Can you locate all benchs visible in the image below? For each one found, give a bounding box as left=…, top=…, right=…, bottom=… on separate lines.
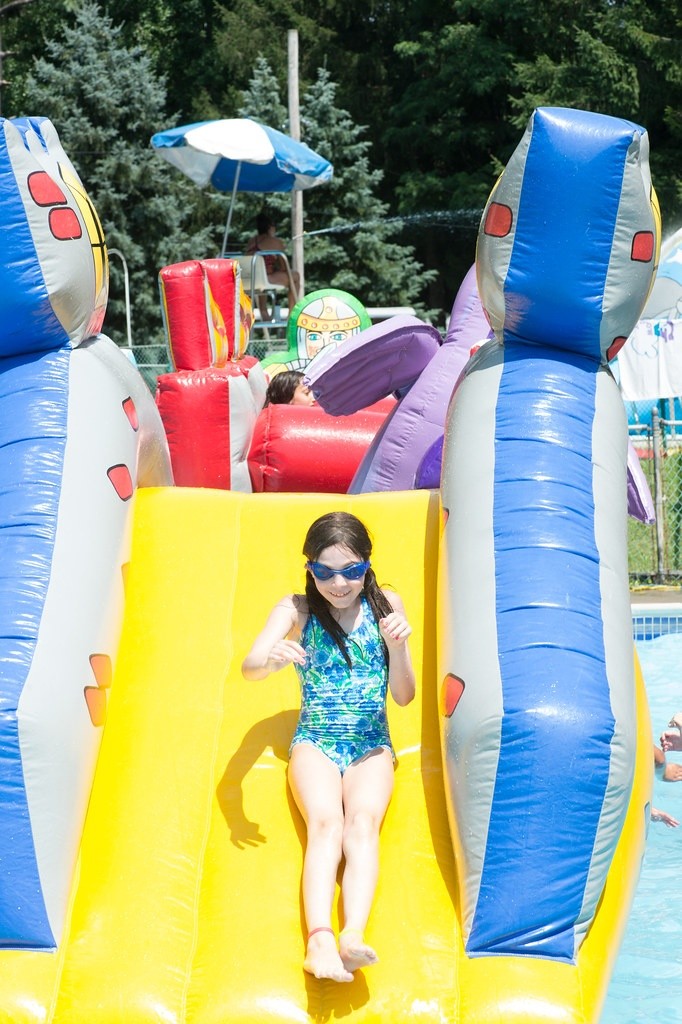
left=220, top=252, right=288, bottom=321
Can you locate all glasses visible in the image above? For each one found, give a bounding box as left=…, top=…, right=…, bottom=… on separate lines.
left=307, top=560, right=372, bottom=582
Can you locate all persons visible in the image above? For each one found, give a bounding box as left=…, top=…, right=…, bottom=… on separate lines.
left=262, top=370, right=315, bottom=407
left=246, top=215, right=301, bottom=338
left=650, top=714, right=682, bottom=828
left=242, top=511, right=415, bottom=982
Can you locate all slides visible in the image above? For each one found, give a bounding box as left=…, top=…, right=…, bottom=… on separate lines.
left=0, top=486, right=654, bottom=1024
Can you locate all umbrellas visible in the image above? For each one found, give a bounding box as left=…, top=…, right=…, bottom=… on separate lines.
left=151, top=118, right=334, bottom=258
